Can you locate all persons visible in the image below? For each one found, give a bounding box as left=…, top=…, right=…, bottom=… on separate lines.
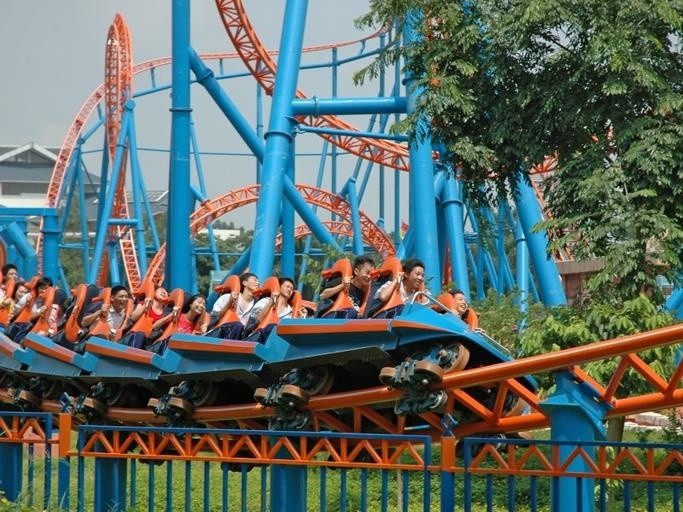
left=1, top=256, right=468, bottom=354
